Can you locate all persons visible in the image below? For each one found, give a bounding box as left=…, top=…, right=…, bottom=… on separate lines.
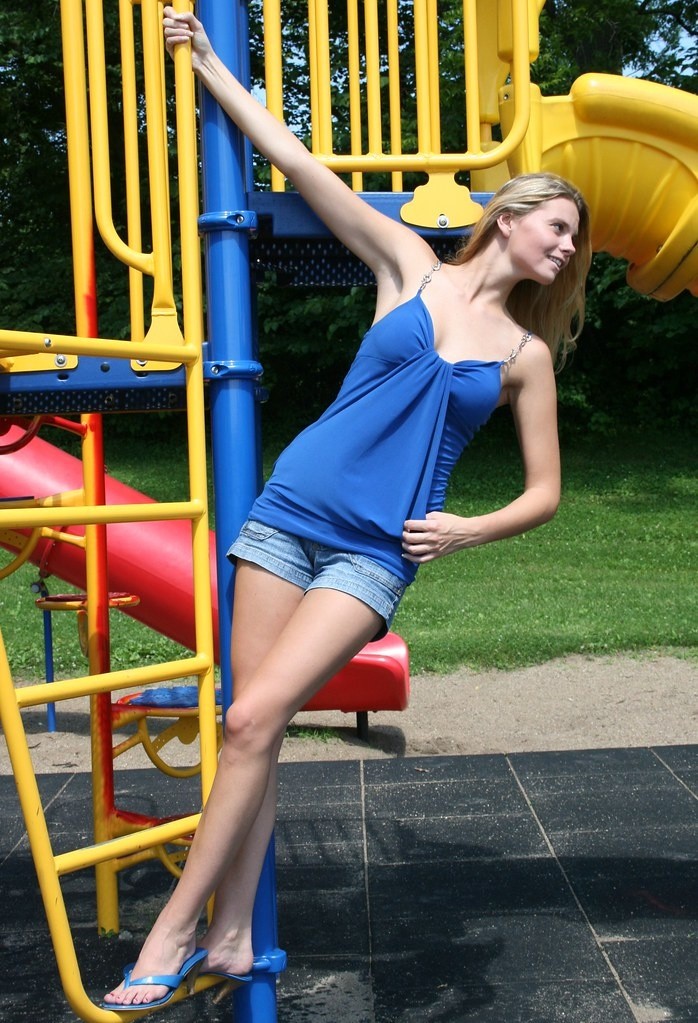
left=97, top=6, right=590, bottom=1010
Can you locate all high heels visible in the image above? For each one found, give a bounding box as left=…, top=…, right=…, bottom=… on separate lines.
left=100, top=945, right=210, bottom=1010
left=185, top=961, right=253, bottom=1005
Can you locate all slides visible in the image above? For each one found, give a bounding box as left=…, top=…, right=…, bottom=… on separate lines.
left=0, top=423, right=411, bottom=709
left=502, top=72, right=698, bottom=302
